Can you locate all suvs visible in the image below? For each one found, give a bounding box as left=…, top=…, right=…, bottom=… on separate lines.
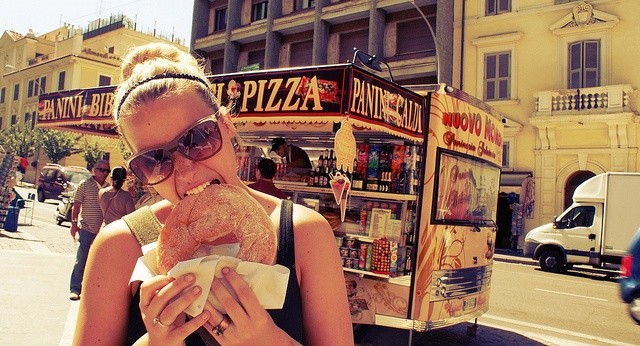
left=37, top=163, right=96, bottom=203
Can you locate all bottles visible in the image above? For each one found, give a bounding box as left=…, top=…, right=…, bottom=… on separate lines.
left=360, top=207, right=367, bottom=231
left=333, top=153, right=336, bottom=169
left=353, top=258, right=359, bottom=269
left=365, top=245, right=372, bottom=272
left=328, top=150, right=333, bottom=169
left=399, top=166, right=406, bottom=193
left=379, top=172, right=385, bottom=192
left=341, top=257, right=346, bottom=268
left=346, top=258, right=353, bottom=269
left=340, top=247, right=350, bottom=257
left=319, top=168, right=323, bottom=186
left=366, top=177, right=379, bottom=191
left=385, top=171, right=391, bottom=193
left=346, top=238, right=354, bottom=248
left=359, top=244, right=367, bottom=270
left=406, top=165, right=415, bottom=194
left=416, top=145, right=420, bottom=169
left=336, top=237, right=344, bottom=247
left=382, top=172, right=388, bottom=192
left=414, top=176, right=418, bottom=191
left=352, top=172, right=364, bottom=191
left=323, top=167, right=328, bottom=186
left=315, top=167, right=319, bottom=186
left=323, top=149, right=328, bottom=169
left=318, top=150, right=323, bottom=169
left=309, top=166, right=314, bottom=186
left=391, top=170, right=398, bottom=193
left=410, top=147, right=416, bottom=170
left=403, top=146, right=410, bottom=170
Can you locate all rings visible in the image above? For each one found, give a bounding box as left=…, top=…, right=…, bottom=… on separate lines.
left=153, top=317, right=170, bottom=329
left=212, top=316, right=229, bottom=335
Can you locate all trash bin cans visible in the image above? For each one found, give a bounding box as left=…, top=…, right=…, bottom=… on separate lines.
left=4, top=206, right=20, bottom=232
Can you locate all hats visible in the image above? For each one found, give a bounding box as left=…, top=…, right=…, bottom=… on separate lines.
left=271, top=138, right=285, bottom=151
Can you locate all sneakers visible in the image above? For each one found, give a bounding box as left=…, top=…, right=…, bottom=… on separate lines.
left=69, top=291, right=80, bottom=299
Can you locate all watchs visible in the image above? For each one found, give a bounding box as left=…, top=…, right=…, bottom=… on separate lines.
left=72, top=219, right=78, bottom=223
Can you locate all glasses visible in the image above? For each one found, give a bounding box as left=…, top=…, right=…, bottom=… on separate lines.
left=96, top=168, right=111, bottom=173
left=129, top=110, right=223, bottom=186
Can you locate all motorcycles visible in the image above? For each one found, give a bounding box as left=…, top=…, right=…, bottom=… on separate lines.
left=56, top=179, right=86, bottom=227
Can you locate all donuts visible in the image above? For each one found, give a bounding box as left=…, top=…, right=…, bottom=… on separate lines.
left=158, top=183, right=277, bottom=285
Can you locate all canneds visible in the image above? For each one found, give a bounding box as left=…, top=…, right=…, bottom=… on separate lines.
left=353, top=259, right=359, bottom=269
left=340, top=258, right=345, bottom=267
left=347, top=258, right=353, bottom=268
left=335, top=238, right=342, bottom=248
left=340, top=248, right=349, bottom=257
left=347, top=238, right=355, bottom=248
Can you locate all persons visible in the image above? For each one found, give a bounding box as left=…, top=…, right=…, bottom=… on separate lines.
left=69, top=160, right=111, bottom=300
left=97, top=167, right=136, bottom=226
left=269, top=138, right=312, bottom=169
left=246, top=158, right=287, bottom=200
left=72, top=42, right=354, bottom=346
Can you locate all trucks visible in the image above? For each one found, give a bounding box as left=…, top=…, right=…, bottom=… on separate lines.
left=523, top=172, right=639, bottom=279
